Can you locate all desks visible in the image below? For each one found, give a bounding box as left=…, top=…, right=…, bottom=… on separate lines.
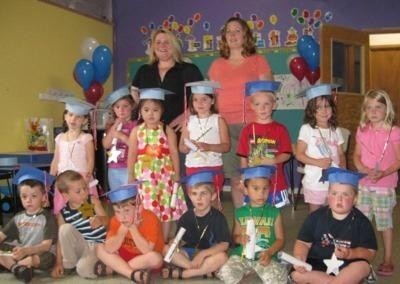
left=0, top=164, right=51, bottom=226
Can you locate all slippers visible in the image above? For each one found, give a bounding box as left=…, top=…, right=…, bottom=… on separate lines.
left=376, top=258, right=395, bottom=276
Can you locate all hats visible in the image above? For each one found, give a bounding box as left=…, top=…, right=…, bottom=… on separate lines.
left=99, top=184, right=140, bottom=225
left=244, top=80, right=282, bottom=95
left=104, top=85, right=130, bottom=106
left=294, top=83, right=342, bottom=109
left=177, top=170, right=224, bottom=211
left=236, top=165, right=277, bottom=206
left=57, top=96, right=97, bottom=151
left=134, top=87, right=175, bottom=120
left=11, top=166, right=55, bottom=209
left=318, top=167, right=368, bottom=184
left=182, top=80, right=222, bottom=126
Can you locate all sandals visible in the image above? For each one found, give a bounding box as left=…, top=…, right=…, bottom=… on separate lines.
left=11, top=265, right=34, bottom=284
left=129, top=268, right=151, bottom=284
left=160, top=265, right=184, bottom=280
left=95, top=260, right=117, bottom=277
left=202, top=272, right=217, bottom=279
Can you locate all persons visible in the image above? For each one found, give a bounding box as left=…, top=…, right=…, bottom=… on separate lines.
left=0, top=79, right=400, bottom=284
left=207, top=17, right=275, bottom=249
left=130, top=28, right=214, bottom=209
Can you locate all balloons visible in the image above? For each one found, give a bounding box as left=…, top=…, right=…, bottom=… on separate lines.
left=73, top=38, right=114, bottom=104
left=290, top=35, right=321, bottom=85
